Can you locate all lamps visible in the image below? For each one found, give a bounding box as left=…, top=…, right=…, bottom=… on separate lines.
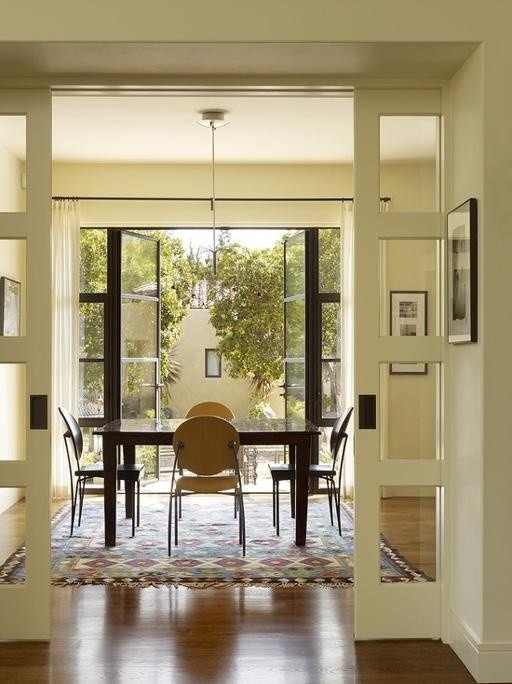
left=195, top=108, right=231, bottom=281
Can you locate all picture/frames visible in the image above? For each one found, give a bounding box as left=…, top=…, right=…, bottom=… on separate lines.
left=0, top=276, right=22, bottom=339
left=443, top=196, right=482, bottom=349
left=389, top=290, right=429, bottom=376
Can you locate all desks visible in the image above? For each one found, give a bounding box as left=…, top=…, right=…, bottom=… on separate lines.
left=86, top=415, right=323, bottom=551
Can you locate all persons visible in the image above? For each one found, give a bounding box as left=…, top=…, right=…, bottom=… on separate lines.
left=451, top=232, right=467, bottom=322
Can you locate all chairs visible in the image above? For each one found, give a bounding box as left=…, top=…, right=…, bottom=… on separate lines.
left=165, top=411, right=249, bottom=560
left=176, top=398, right=247, bottom=523
left=55, top=401, right=147, bottom=541
left=267, top=403, right=356, bottom=540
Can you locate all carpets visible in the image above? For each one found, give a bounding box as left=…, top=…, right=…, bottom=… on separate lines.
left=0, top=491, right=432, bottom=596
